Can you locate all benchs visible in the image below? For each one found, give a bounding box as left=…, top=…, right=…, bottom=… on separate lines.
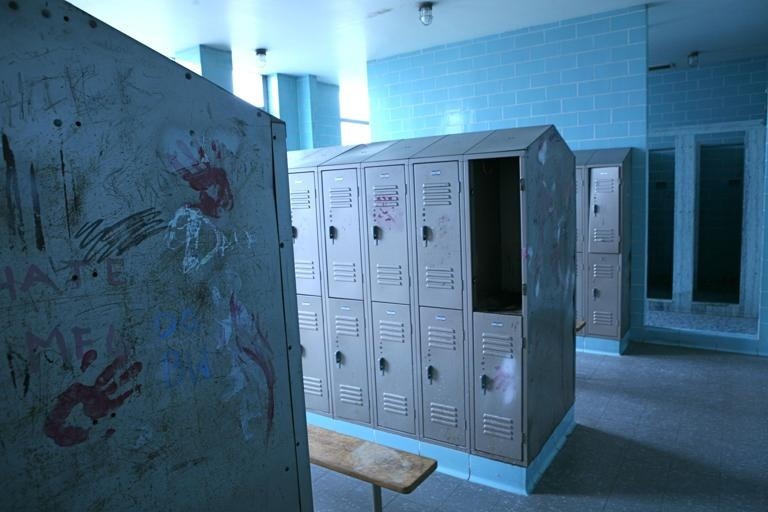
left=302, top=423, right=440, bottom=512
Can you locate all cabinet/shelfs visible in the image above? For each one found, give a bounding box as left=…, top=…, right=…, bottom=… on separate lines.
left=469, top=312, right=585, bottom=466
left=572, top=148, right=599, bottom=252
left=323, top=298, right=374, bottom=431
left=584, top=251, right=632, bottom=341
left=297, top=295, right=333, bottom=421
left=318, top=138, right=404, bottom=297
left=360, top=133, right=449, bottom=305
left=409, top=129, right=497, bottom=308
left=585, top=147, right=633, bottom=254
left=415, top=304, right=471, bottom=455
left=368, top=301, right=418, bottom=439
left=286, top=143, right=360, bottom=295
left=463, top=124, right=578, bottom=316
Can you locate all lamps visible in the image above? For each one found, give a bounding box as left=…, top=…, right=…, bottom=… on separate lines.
left=688, top=52, right=698, bottom=68
left=254, top=48, right=267, bottom=68
left=419, top=2, right=433, bottom=26
